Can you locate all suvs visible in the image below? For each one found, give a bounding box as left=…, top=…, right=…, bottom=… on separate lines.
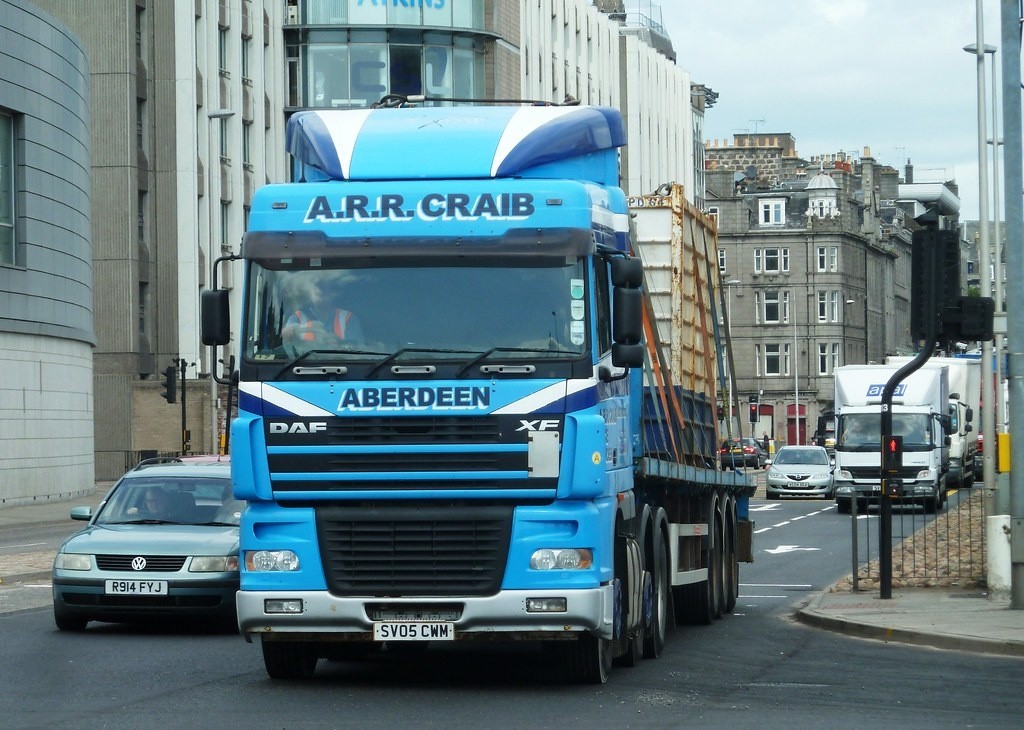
left=51, top=456, right=247, bottom=632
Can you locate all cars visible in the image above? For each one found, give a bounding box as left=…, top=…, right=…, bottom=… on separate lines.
left=171, top=456, right=231, bottom=505
left=719, top=437, right=768, bottom=470
left=811, top=428, right=836, bottom=456
left=764, top=445, right=836, bottom=500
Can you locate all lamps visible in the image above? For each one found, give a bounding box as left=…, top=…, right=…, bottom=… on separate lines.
left=207, top=108, right=236, bottom=121
left=845, top=299, right=855, bottom=306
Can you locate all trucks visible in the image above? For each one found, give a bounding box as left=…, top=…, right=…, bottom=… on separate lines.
left=833, top=364, right=952, bottom=514
left=951, top=354, right=1009, bottom=482
left=885, top=356, right=982, bottom=489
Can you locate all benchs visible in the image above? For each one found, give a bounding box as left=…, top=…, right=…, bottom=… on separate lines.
left=332, top=99, right=367, bottom=107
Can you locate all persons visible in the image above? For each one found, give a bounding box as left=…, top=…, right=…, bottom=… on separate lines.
left=762, top=430, right=769, bottom=444
left=213, top=484, right=247, bottom=524
left=780, top=450, right=823, bottom=465
left=281, top=275, right=367, bottom=350
left=848, top=420, right=868, bottom=441
left=127, top=487, right=178, bottom=522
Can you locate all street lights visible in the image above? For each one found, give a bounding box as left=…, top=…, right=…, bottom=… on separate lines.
left=208, top=108, right=236, bottom=456
left=964, top=43, right=1006, bottom=433
left=727, top=279, right=741, bottom=435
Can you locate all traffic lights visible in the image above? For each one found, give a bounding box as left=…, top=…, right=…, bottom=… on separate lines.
left=185, top=430, right=191, bottom=442
left=882, top=435, right=902, bottom=472
left=160, top=366, right=175, bottom=403
left=750, top=403, right=759, bottom=423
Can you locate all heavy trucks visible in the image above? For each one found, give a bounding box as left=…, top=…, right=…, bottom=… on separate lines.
left=203, top=92, right=758, bottom=686
